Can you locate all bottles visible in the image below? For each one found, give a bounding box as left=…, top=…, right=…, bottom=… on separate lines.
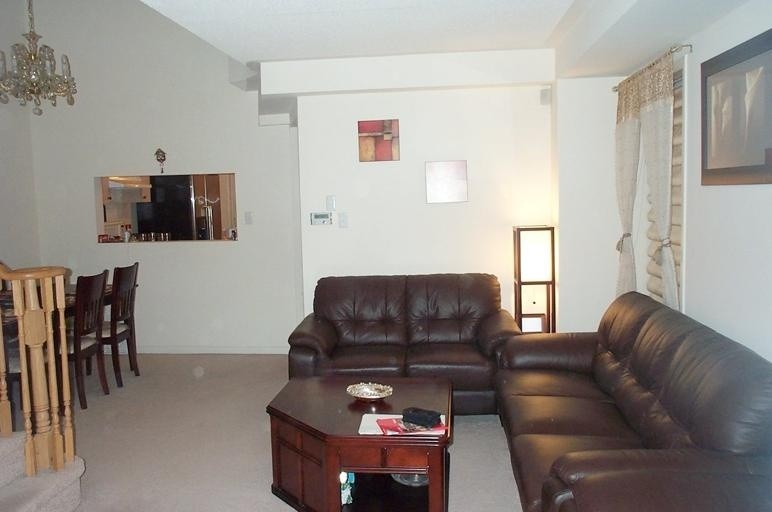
left=121, top=224, right=171, bottom=242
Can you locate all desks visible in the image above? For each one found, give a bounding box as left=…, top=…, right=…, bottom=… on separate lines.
left=0, top=283, right=139, bottom=337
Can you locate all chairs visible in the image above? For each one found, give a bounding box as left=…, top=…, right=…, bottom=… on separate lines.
left=6, top=278, right=66, bottom=432
left=55, top=269, right=110, bottom=410
left=87, top=262, right=140, bottom=387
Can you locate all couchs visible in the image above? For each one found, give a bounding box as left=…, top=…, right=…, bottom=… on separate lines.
left=493, top=291, right=772, bottom=512
left=288, top=273, right=523, bottom=416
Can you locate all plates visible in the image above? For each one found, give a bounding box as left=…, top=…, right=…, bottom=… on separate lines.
left=345, top=383, right=393, bottom=402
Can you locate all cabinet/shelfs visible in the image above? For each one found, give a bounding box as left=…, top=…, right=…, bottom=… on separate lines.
left=193, top=175, right=234, bottom=239
left=101, top=176, right=151, bottom=204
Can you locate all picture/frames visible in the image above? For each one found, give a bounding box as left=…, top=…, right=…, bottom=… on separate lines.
left=701, top=28, right=772, bottom=185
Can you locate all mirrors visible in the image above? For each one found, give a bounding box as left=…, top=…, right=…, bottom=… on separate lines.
left=93, top=173, right=238, bottom=243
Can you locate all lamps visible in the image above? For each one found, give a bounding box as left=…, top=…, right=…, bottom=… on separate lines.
left=513, top=225, right=556, bottom=334
left=0, top=0, right=77, bottom=115
